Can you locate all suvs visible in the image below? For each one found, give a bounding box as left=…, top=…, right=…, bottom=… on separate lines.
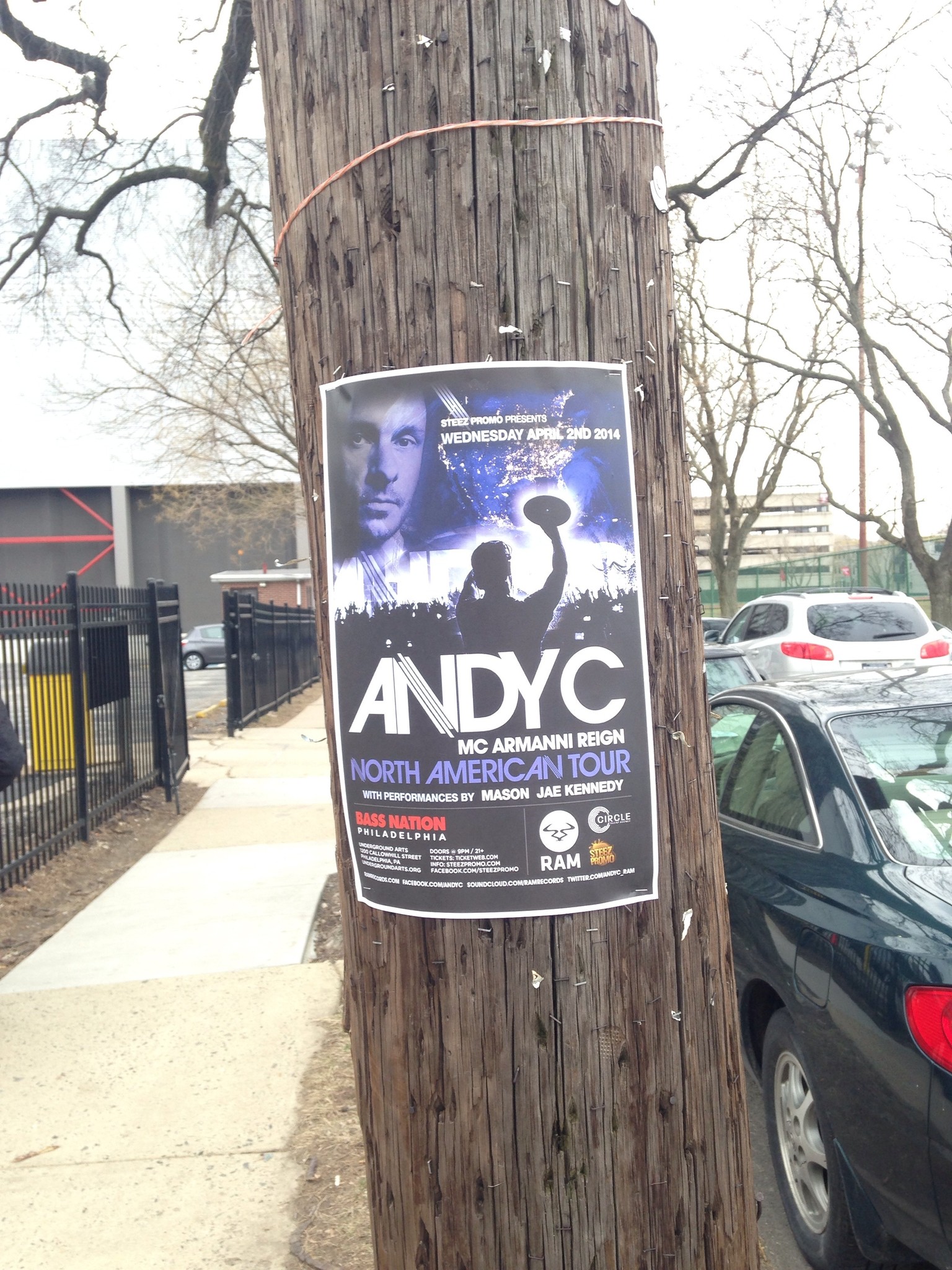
left=718, top=586, right=951, bottom=681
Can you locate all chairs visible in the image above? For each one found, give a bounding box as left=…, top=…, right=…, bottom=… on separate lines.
left=817, top=616, right=856, bottom=641
left=750, top=744, right=808, bottom=833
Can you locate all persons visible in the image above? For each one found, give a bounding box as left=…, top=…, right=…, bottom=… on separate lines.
left=329, top=384, right=427, bottom=614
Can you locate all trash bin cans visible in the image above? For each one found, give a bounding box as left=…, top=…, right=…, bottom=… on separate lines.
left=22, top=638, right=97, bottom=772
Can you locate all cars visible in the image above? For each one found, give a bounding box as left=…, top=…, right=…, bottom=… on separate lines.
left=704, top=665, right=952, bottom=1270
left=696, top=617, right=763, bottom=695
left=179, top=624, right=227, bottom=672
